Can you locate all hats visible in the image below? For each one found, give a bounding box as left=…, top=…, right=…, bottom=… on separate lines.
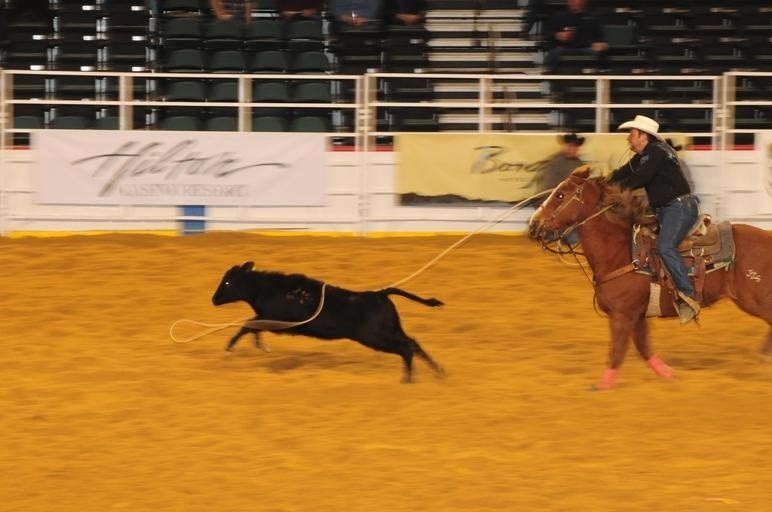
left=558, top=132, right=585, bottom=146
left=617, top=114, right=664, bottom=142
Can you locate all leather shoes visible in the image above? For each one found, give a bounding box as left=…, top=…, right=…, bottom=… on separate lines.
left=678, top=300, right=695, bottom=323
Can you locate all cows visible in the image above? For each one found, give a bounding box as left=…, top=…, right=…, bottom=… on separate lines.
left=211, top=261, right=445, bottom=385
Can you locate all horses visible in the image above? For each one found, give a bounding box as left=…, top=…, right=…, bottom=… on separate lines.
left=529, top=165, right=772, bottom=389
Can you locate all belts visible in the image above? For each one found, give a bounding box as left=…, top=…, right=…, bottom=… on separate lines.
left=666, top=194, right=691, bottom=207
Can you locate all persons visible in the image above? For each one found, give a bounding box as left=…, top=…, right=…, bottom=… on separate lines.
left=664, top=138, right=695, bottom=195
left=603, top=113, right=700, bottom=325
left=534, top=132, right=586, bottom=244
left=542, top=1, right=616, bottom=74
left=206, top=1, right=425, bottom=28
